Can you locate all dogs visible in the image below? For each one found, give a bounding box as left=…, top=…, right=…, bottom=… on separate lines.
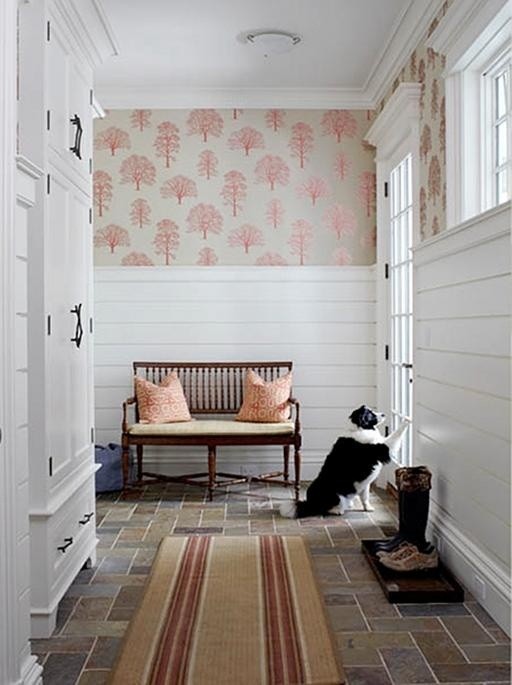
left=278, top=405, right=409, bottom=519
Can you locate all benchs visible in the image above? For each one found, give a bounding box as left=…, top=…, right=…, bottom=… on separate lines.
left=119, top=360, right=302, bottom=502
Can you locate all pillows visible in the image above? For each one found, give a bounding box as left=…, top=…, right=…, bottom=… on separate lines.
left=135, top=373, right=190, bottom=426
left=234, top=365, right=294, bottom=424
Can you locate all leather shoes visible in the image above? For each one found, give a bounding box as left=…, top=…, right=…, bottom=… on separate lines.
left=375, top=540, right=432, bottom=562
left=377, top=544, right=440, bottom=572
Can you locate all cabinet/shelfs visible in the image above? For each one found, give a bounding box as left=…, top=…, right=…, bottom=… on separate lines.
left=30, top=157, right=101, bottom=641
left=46, top=0, right=117, bottom=197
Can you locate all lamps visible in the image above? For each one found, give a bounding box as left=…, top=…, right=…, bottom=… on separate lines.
left=246, top=33, right=300, bottom=59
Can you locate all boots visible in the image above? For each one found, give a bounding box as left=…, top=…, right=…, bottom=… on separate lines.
left=373, top=464, right=439, bottom=554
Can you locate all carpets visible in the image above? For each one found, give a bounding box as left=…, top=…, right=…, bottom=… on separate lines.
left=108, top=533, right=349, bottom=685
